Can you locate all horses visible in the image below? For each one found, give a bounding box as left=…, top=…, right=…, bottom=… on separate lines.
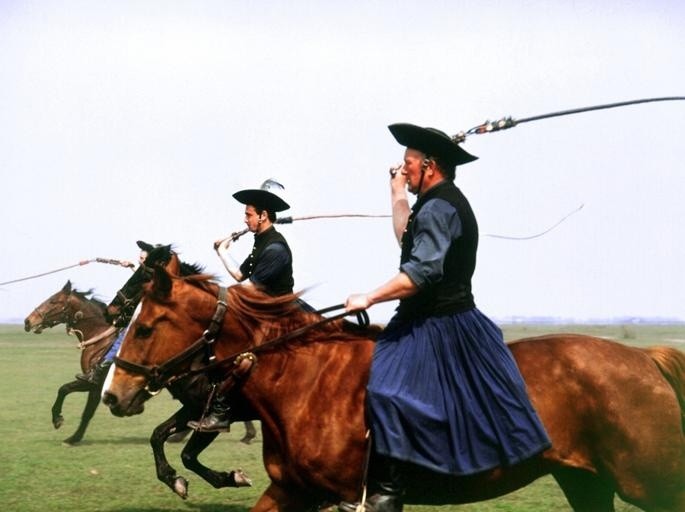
left=23, top=280, right=258, bottom=450
left=102, top=239, right=262, bottom=500
left=99, top=264, right=685, bottom=512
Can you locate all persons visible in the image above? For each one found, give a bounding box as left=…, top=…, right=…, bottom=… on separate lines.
left=336, top=122, right=554, bottom=512
left=75, top=246, right=150, bottom=387
left=185, top=189, right=293, bottom=434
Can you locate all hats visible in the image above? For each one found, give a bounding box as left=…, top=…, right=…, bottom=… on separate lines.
left=136, top=240, right=163, bottom=254
left=232, top=178, right=290, bottom=212
left=388, top=115, right=514, bottom=166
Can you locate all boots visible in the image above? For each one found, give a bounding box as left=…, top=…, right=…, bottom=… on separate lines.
left=187, top=389, right=230, bottom=433
left=338, top=456, right=412, bottom=512
